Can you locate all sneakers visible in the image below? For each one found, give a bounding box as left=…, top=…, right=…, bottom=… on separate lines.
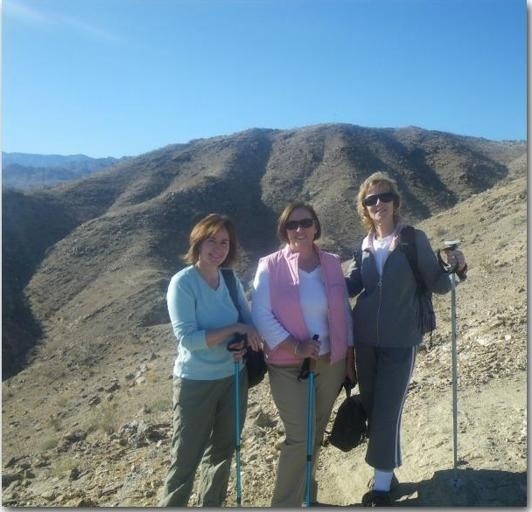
left=367, top=473, right=400, bottom=506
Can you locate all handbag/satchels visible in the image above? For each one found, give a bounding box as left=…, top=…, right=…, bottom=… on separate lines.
left=330, top=394, right=368, bottom=451
left=248, top=345, right=267, bottom=387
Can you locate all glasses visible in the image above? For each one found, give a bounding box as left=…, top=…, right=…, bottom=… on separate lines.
left=362, top=192, right=394, bottom=205
left=285, top=219, right=313, bottom=230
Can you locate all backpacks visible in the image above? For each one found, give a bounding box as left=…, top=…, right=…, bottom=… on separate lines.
left=354, top=226, right=438, bottom=331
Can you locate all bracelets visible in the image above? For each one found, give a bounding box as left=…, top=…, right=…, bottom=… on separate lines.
left=292, top=340, right=301, bottom=356
left=460, top=264, right=467, bottom=274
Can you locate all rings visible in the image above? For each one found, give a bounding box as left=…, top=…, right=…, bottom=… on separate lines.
left=450, top=255, right=456, bottom=260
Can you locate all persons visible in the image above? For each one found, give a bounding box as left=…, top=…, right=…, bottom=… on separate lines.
left=158, top=213, right=265, bottom=507
left=338, top=168, right=468, bottom=508
left=249, top=199, right=359, bottom=508
